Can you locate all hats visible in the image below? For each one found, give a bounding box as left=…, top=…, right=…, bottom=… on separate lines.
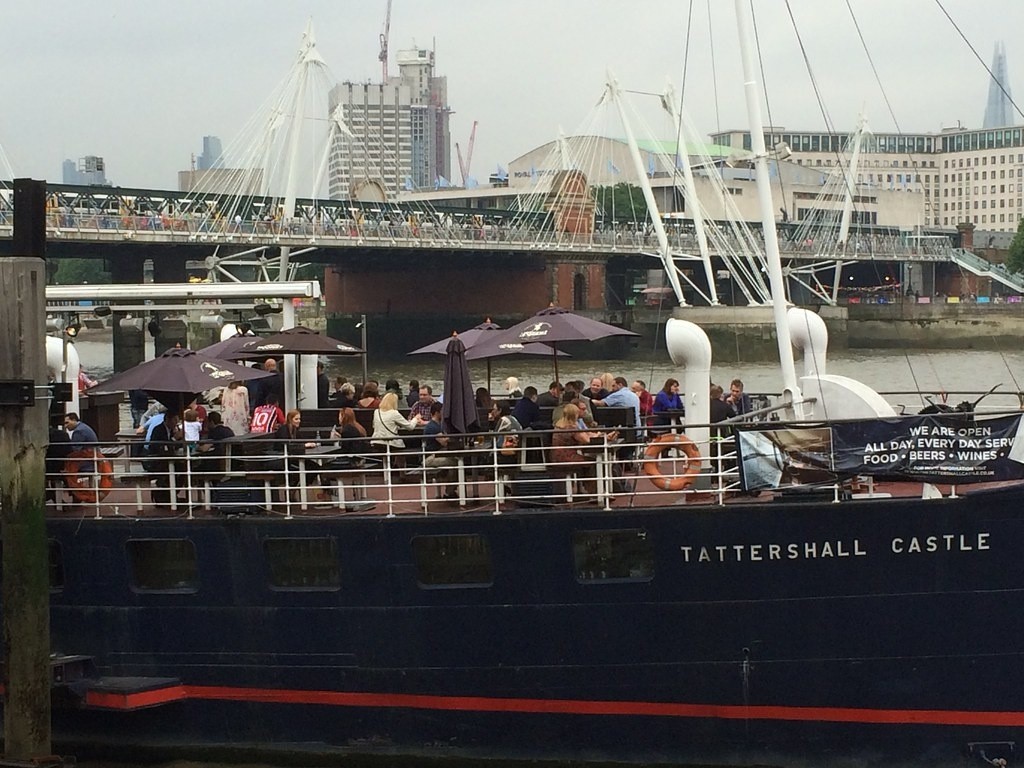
left=710, top=385, right=723, bottom=399
left=562, top=391, right=576, bottom=402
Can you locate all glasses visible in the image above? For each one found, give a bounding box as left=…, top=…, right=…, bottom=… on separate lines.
left=343, top=407, right=346, bottom=413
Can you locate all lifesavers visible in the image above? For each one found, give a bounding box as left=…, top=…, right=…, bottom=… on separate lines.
left=643, top=434, right=702, bottom=491
left=65, top=450, right=113, bottom=503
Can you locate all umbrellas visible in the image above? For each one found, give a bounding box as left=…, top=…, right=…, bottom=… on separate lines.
left=458, top=302, right=644, bottom=404
left=193, top=322, right=285, bottom=368
left=405, top=316, right=575, bottom=396
left=442, top=330, right=481, bottom=447
left=233, top=321, right=368, bottom=408
left=81, top=340, right=277, bottom=458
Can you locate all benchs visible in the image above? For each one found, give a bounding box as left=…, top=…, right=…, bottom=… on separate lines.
left=183, top=443, right=226, bottom=511
left=120, top=470, right=148, bottom=511
left=321, top=440, right=379, bottom=510
left=482, top=434, right=522, bottom=505
left=47, top=471, right=94, bottom=512
left=241, top=444, right=279, bottom=514
left=543, top=434, right=591, bottom=503
left=395, top=453, right=445, bottom=511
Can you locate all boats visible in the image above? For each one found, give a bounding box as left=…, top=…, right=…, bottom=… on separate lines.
left=0, top=3, right=1024, bottom=768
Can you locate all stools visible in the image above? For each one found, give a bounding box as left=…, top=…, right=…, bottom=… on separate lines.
left=100, top=448, right=124, bottom=481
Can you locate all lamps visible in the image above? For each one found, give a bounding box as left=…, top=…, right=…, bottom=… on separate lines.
left=235, top=323, right=251, bottom=337
left=163, top=315, right=190, bottom=329
left=199, top=315, right=225, bottom=328
left=83, top=317, right=108, bottom=330
left=66, top=323, right=82, bottom=338
left=46, top=318, right=65, bottom=331
left=119, top=318, right=143, bottom=334
left=94, top=306, right=112, bottom=317
left=248, top=316, right=272, bottom=330
left=148, top=321, right=161, bottom=337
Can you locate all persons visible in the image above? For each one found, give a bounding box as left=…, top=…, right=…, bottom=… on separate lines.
left=369, top=392, right=423, bottom=483
left=406, top=385, right=439, bottom=427
left=244, top=359, right=284, bottom=413
left=318, top=361, right=420, bottom=409
left=651, top=378, right=684, bottom=459
left=710, top=378, right=750, bottom=471
left=137, top=397, right=212, bottom=455
left=420, top=402, right=459, bottom=499
left=248, top=392, right=286, bottom=435
left=569, top=398, right=631, bottom=493
left=63, top=412, right=99, bottom=510
left=473, top=372, right=654, bottom=476
left=546, top=402, right=616, bottom=502
left=147, top=408, right=186, bottom=496
left=314, top=406, right=369, bottom=509
left=271, top=408, right=321, bottom=501
left=192, top=412, right=235, bottom=495
left=221, top=380, right=251, bottom=439
left=78, top=363, right=99, bottom=396
left=45, top=415, right=73, bottom=504
left=482, top=400, right=523, bottom=498
left=47, top=365, right=57, bottom=412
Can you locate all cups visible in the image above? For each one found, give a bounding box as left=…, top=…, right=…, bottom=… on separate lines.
left=478, top=436, right=484, bottom=445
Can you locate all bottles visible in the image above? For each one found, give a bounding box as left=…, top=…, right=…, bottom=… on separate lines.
left=315, top=431, right=322, bottom=447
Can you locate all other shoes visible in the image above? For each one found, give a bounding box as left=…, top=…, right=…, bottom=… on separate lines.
left=316, top=493, right=331, bottom=499
left=444, top=495, right=458, bottom=500
left=284, top=490, right=298, bottom=506
left=504, top=485, right=511, bottom=494
left=313, top=502, right=334, bottom=510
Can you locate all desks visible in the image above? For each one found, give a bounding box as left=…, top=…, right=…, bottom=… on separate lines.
left=435, top=443, right=492, bottom=507
left=653, top=411, right=684, bottom=457
left=114, top=428, right=146, bottom=473
left=581, top=438, right=626, bottom=508
left=146, top=448, right=215, bottom=511
left=298, top=427, right=340, bottom=446
left=279, top=446, right=341, bottom=511
